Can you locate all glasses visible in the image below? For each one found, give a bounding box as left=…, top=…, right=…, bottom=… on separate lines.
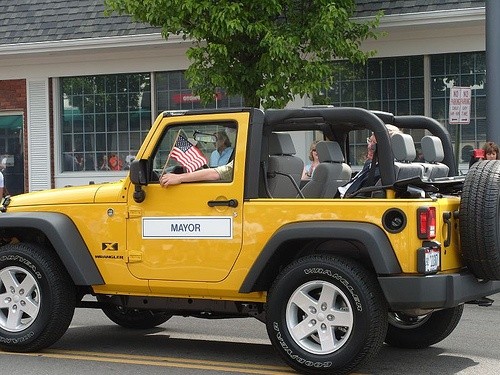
left=367, top=138, right=377, bottom=145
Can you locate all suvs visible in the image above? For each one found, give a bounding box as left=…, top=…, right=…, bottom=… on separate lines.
left=0, top=104, right=500, bottom=375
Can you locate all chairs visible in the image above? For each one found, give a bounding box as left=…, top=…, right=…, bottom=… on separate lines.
left=268, top=133, right=305, bottom=198
left=295, top=141, right=352, bottom=200
left=420, top=135, right=450, bottom=178
left=371, top=133, right=425, bottom=198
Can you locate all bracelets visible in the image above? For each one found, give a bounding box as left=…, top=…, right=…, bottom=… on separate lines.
left=108, top=167, right=111, bottom=170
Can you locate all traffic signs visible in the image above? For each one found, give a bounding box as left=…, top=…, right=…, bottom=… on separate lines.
left=449, top=86, right=472, bottom=125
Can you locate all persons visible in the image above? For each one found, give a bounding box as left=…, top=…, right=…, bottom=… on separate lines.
left=2, top=155, right=8, bottom=168
left=74, top=150, right=95, bottom=171
left=363, top=123, right=400, bottom=171
left=0, top=171, right=4, bottom=205
left=301, top=140, right=319, bottom=179
left=160, top=158, right=234, bottom=190
left=126, top=152, right=133, bottom=169
left=469, top=142, right=499, bottom=170
left=100, top=155, right=124, bottom=170
left=209, top=132, right=234, bottom=168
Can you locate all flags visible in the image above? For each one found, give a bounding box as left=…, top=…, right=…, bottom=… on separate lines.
left=169, top=134, right=207, bottom=173
